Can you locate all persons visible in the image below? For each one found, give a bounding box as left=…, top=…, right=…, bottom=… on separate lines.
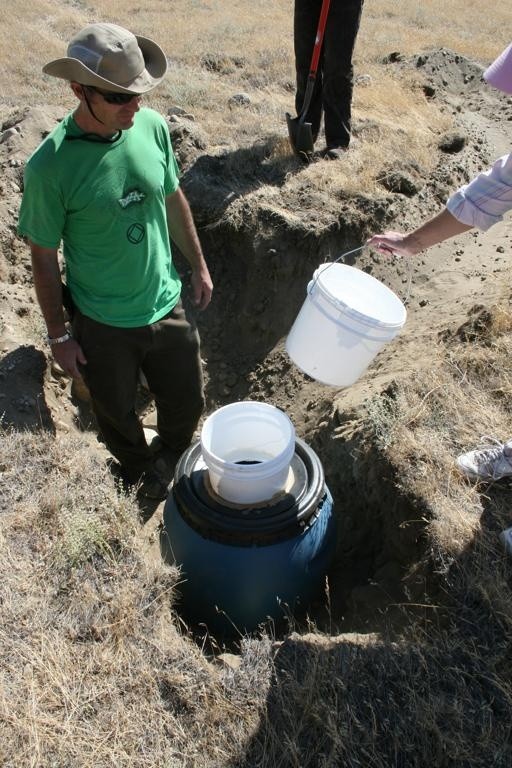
left=292, top=0, right=365, bottom=160
left=365, top=41, right=512, bottom=554
left=14, top=20, right=215, bottom=503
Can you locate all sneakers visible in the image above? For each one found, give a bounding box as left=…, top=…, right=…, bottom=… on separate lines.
left=321, top=144, right=347, bottom=162
left=131, top=436, right=194, bottom=502
left=454, top=440, right=512, bottom=485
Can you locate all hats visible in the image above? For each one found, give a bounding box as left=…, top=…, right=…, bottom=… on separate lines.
left=42, top=22, right=168, bottom=95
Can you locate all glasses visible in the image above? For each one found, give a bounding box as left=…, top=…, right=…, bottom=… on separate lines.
left=87, top=86, right=142, bottom=105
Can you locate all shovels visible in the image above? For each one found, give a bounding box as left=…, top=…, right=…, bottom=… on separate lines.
left=286, top=0, right=330, bottom=164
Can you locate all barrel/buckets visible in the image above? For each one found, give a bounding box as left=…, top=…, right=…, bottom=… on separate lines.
left=198, top=397, right=298, bottom=501
left=159, top=437, right=337, bottom=637
left=284, top=241, right=413, bottom=390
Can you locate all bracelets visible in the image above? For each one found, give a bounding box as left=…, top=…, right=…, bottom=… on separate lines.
left=46, top=329, right=71, bottom=345
